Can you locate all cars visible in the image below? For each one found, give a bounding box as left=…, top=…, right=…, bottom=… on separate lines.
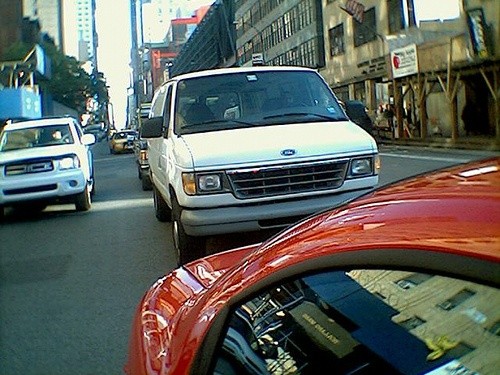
left=147, top=66, right=381, bottom=269
left=1, top=116, right=97, bottom=215
left=125, top=159, right=500, bottom=374
left=109, top=131, right=139, bottom=152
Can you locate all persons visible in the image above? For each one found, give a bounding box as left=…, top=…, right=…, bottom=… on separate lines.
left=52, top=128, right=70, bottom=143
left=276, top=91, right=294, bottom=109
left=379, top=103, right=422, bottom=138
